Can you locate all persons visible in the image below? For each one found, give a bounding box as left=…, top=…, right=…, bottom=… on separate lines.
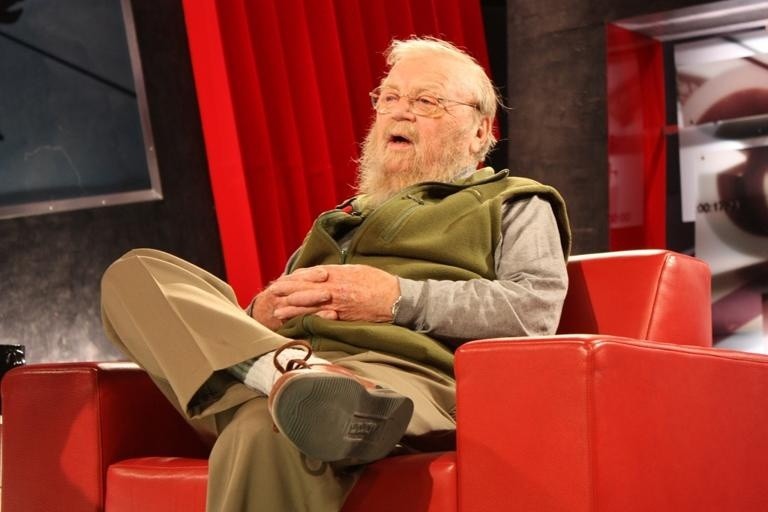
left=99, top=37, right=572, bottom=511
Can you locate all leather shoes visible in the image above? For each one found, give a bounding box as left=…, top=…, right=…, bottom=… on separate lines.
left=266, top=361, right=413, bottom=467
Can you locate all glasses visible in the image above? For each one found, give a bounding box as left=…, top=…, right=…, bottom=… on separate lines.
left=369, top=86, right=480, bottom=118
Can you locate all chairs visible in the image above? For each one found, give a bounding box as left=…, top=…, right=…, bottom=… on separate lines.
left=1, top=248, right=768, bottom=508
